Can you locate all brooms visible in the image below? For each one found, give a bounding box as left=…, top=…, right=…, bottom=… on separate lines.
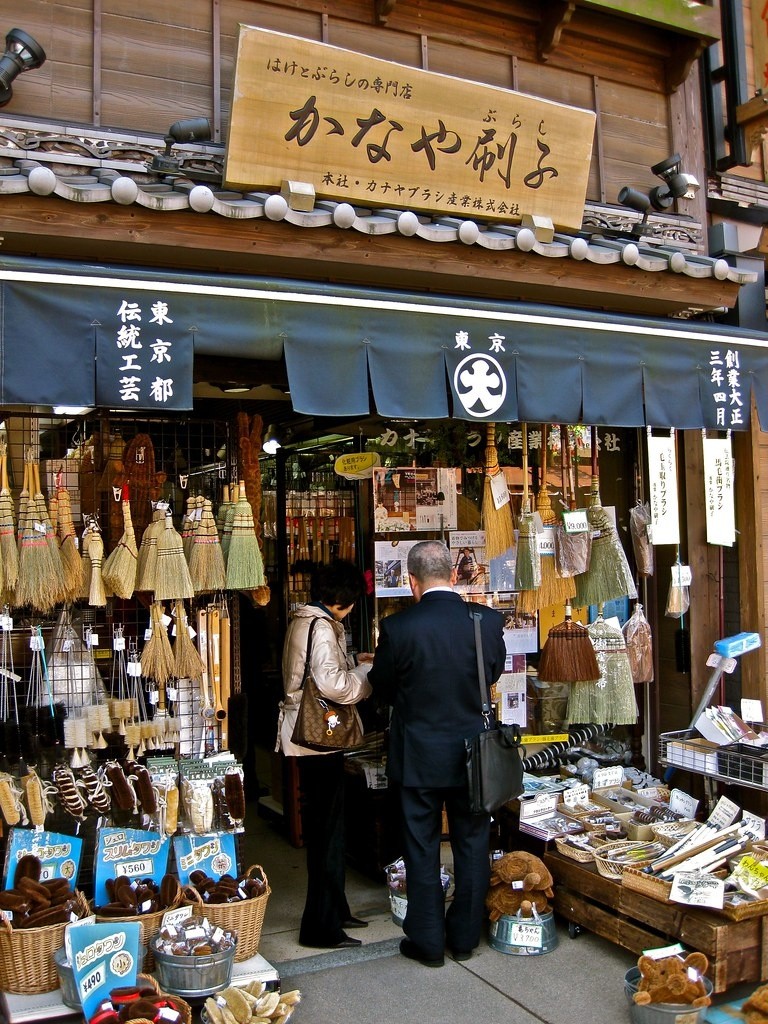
left=0, top=420, right=655, bottom=728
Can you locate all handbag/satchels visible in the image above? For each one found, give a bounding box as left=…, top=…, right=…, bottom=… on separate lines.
left=465, top=720, right=526, bottom=816
left=291, top=617, right=367, bottom=752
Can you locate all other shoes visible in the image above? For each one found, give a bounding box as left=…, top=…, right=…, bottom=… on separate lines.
left=341, top=917, right=368, bottom=928
left=399, top=938, right=444, bottom=966
left=332, top=937, right=361, bottom=948
left=444, top=940, right=472, bottom=960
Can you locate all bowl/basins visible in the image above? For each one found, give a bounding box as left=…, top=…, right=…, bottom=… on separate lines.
left=389, top=882, right=451, bottom=927
left=625, top=966, right=713, bottom=1024
left=488, top=907, right=560, bottom=956
left=150, top=933, right=239, bottom=997
left=54, top=945, right=147, bottom=1012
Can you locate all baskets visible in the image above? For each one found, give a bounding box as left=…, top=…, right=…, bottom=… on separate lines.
left=0, top=890, right=92, bottom=995
left=75, top=873, right=182, bottom=974
left=553, top=821, right=768, bottom=922
left=182, top=864, right=271, bottom=963
left=716, top=743, right=768, bottom=785
left=82, top=974, right=191, bottom=1024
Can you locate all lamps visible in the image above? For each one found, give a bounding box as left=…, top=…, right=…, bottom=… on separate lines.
left=647, top=153, right=686, bottom=210
left=617, top=186, right=655, bottom=238
left=144, top=117, right=213, bottom=179
left=216, top=445, right=229, bottom=460
left=0, top=26, right=47, bottom=107
left=262, top=424, right=284, bottom=455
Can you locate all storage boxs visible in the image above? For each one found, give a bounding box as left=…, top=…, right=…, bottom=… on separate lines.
left=667, top=739, right=720, bottom=772
left=695, top=711, right=759, bottom=746
left=594, top=787, right=663, bottom=814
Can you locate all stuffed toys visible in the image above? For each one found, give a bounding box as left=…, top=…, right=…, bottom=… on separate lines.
left=488, top=850, right=554, bottom=924
left=632, top=952, right=768, bottom=1024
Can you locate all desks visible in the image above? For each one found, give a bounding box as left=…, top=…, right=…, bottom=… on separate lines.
left=545, top=851, right=768, bottom=994
left=2, top=951, right=281, bottom=1024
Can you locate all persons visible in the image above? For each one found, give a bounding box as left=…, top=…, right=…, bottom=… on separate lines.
left=374, top=471, right=536, bottom=629
left=366, top=540, right=506, bottom=967
left=272, top=557, right=373, bottom=947
